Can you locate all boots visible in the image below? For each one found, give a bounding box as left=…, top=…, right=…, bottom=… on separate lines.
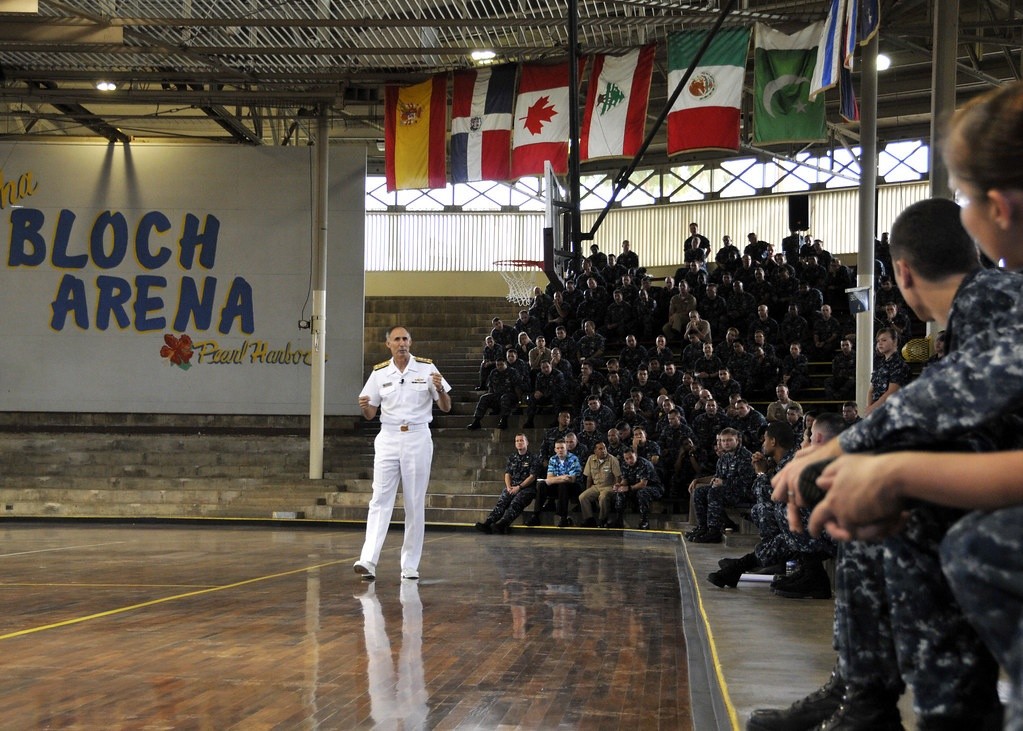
left=745, top=666, right=847, bottom=731
left=638, top=513, right=650, bottom=529
left=813, top=683, right=905, bottom=731
left=660, top=506, right=736, bottom=543
left=769, top=556, right=832, bottom=600
left=707, top=554, right=760, bottom=588
left=607, top=513, right=624, bottom=529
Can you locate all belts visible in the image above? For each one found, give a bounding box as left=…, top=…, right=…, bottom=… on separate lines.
left=382, top=423, right=428, bottom=432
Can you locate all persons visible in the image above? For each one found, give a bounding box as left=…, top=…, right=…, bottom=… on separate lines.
left=352, top=326, right=452, bottom=580
left=468, top=224, right=946, bottom=598
left=742, top=198, right=1023, bottom=731
left=354, top=580, right=429, bottom=730
left=807, top=81, right=1023, bottom=731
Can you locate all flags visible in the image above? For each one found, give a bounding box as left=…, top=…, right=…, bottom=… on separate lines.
left=383, top=75, right=446, bottom=193
left=580, top=45, right=655, bottom=164
left=808, top=0, right=880, bottom=123
left=667, top=25, right=753, bottom=158
left=752, top=18, right=829, bottom=148
left=512, top=58, right=588, bottom=179
left=451, top=63, right=518, bottom=181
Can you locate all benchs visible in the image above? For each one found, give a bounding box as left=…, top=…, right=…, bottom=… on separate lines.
left=0, top=293, right=856, bottom=531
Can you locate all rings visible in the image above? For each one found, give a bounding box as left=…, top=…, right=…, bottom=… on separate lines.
left=787, top=491, right=794, bottom=496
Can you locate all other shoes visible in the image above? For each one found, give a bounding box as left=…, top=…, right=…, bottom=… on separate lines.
left=400, top=579, right=420, bottom=586
left=571, top=503, right=582, bottom=513
left=467, top=378, right=582, bottom=432
left=541, top=501, right=557, bottom=513
left=580, top=517, right=596, bottom=527
left=353, top=581, right=375, bottom=599
left=557, top=518, right=569, bottom=527
left=401, top=569, right=420, bottom=579
left=490, top=521, right=509, bottom=534
left=591, top=502, right=599, bottom=514
left=610, top=501, right=617, bottom=514
left=476, top=519, right=494, bottom=534
left=524, top=514, right=541, bottom=526
left=631, top=498, right=640, bottom=513
left=353, top=561, right=376, bottom=581
left=596, top=519, right=609, bottom=528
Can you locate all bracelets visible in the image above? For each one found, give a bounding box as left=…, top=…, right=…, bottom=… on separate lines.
left=435, top=388, right=444, bottom=393
